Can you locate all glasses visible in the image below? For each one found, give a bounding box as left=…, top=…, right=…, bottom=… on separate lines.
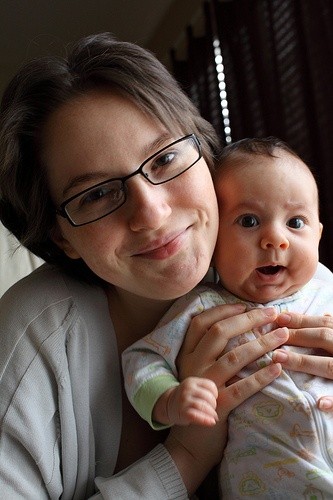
left=51, top=132, right=204, bottom=228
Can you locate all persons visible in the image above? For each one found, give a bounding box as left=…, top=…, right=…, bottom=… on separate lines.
left=0, top=32, right=333, bottom=500
left=120, top=136, right=332, bottom=500
